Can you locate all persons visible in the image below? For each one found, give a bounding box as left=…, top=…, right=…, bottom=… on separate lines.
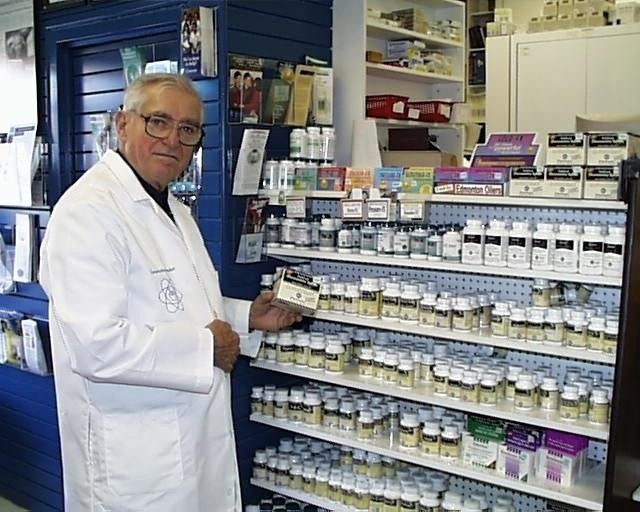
left=38, top=71, right=302, bottom=512
left=228, top=71, right=261, bottom=119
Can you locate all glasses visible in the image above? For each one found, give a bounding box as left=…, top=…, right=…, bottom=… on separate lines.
left=131, top=109, right=206, bottom=147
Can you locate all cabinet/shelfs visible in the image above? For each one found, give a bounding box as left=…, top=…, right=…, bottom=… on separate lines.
left=247, top=1, right=640, bottom=512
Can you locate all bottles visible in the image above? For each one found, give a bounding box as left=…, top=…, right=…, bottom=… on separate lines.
left=244, top=123, right=626, bottom=511
